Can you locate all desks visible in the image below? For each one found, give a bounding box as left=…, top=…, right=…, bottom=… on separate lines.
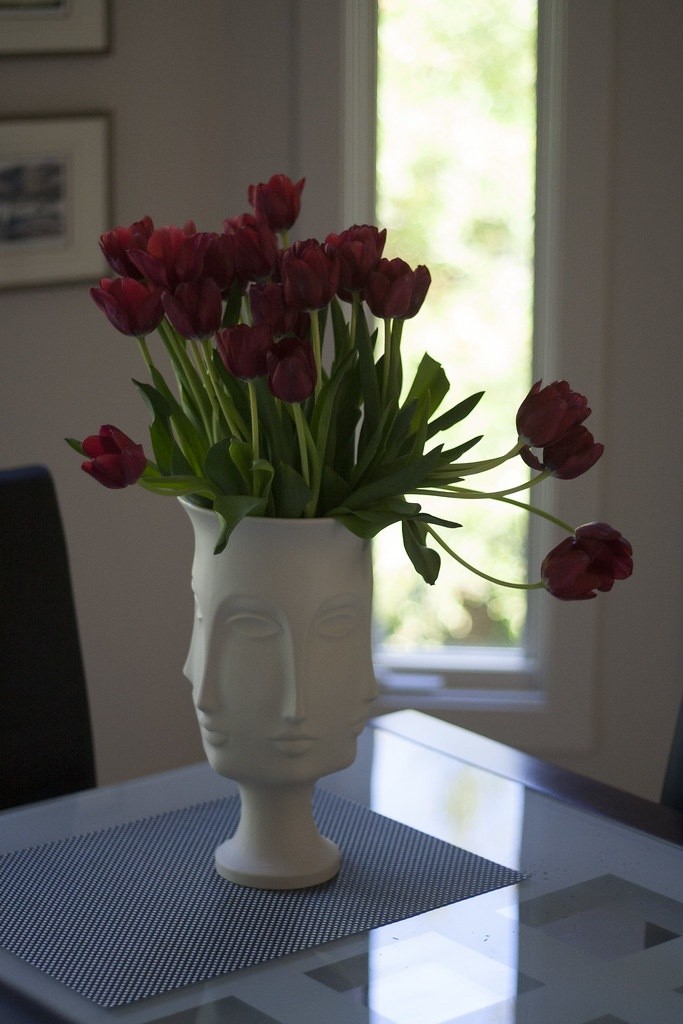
left=0, top=706, right=683, bottom=1024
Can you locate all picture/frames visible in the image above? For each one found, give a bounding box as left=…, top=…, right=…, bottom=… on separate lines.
left=0, top=0, right=115, bottom=60
left=0, top=107, right=117, bottom=295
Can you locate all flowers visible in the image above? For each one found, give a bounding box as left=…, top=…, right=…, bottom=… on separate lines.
left=63, top=176, right=637, bottom=600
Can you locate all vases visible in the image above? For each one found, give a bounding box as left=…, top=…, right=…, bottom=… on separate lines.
left=177, top=498, right=382, bottom=889
